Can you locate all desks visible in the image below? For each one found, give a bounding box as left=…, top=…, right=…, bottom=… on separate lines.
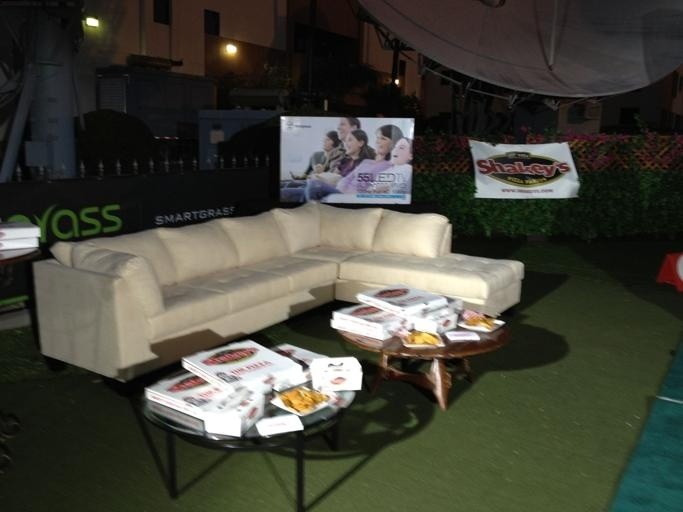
left=0, top=248, right=43, bottom=474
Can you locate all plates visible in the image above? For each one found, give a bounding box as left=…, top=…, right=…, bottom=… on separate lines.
left=457, top=316, right=508, bottom=335
left=270, top=385, right=337, bottom=418
left=399, top=330, right=448, bottom=349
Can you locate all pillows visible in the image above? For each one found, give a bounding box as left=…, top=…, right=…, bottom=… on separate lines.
left=372, top=209, right=448, bottom=259
left=310, top=200, right=383, bottom=250
left=154, top=220, right=240, bottom=280
left=270, top=200, right=320, bottom=253
left=215, top=212, right=290, bottom=266
left=50, top=229, right=178, bottom=286
left=73, top=245, right=165, bottom=312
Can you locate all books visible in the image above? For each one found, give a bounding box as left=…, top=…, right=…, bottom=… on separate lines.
left=327, top=285, right=467, bottom=342
left=143, top=337, right=328, bottom=420
left=0, top=222, right=42, bottom=250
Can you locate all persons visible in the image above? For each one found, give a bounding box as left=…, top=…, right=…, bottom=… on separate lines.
left=293, top=118, right=413, bottom=201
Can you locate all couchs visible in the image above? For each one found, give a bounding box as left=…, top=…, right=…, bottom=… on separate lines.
left=33, top=224, right=526, bottom=390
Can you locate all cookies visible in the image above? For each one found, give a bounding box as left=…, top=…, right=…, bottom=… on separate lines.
left=280, top=387, right=330, bottom=413
left=407, top=330, right=440, bottom=352
left=467, top=316, right=496, bottom=331
